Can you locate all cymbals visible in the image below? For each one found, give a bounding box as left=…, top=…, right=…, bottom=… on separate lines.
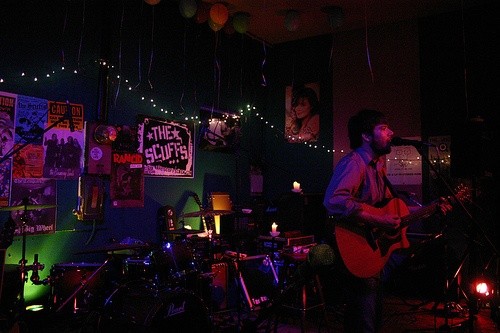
left=179, top=209, right=235, bottom=218
left=163, top=228, right=205, bottom=234
left=88, top=244, right=137, bottom=254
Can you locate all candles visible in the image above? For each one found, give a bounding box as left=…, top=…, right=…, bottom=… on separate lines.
left=272, top=223, right=278, bottom=232
left=293, top=181, right=300, bottom=189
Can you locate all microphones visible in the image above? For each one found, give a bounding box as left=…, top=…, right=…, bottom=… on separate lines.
left=66, top=98, right=74, bottom=132
left=391, top=137, right=436, bottom=147
left=197, top=113, right=210, bottom=145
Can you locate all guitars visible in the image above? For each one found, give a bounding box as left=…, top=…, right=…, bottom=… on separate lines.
left=333, top=183, right=473, bottom=278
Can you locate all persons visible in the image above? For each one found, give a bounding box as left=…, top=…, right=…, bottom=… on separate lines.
left=323, top=108, right=452, bottom=333
left=44, top=133, right=82, bottom=169
left=294, top=87, right=319, bottom=142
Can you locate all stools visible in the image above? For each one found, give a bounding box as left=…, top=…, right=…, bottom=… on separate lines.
left=273, top=252, right=332, bottom=333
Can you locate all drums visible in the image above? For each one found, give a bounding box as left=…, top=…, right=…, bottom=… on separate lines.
left=155, top=238, right=201, bottom=283
left=50, top=262, right=105, bottom=313
left=98, top=279, right=212, bottom=333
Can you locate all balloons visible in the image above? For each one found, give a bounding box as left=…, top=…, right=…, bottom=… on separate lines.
left=146, top=0, right=249, bottom=33
left=284, top=11, right=300, bottom=32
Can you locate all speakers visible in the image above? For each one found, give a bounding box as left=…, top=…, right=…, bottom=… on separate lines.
left=211, top=263, right=230, bottom=314
